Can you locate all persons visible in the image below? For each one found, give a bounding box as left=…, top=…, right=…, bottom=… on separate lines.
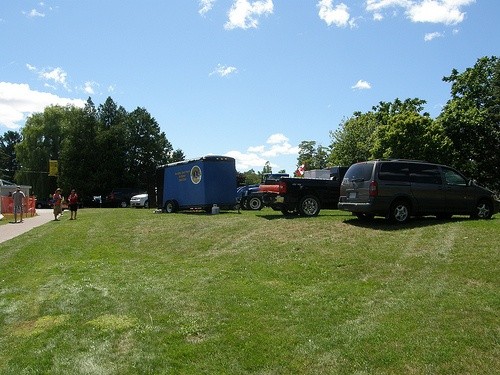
left=53, top=188, right=62, bottom=221
left=12, top=186, right=25, bottom=223
left=68, top=190, right=78, bottom=220
left=110, top=192, right=115, bottom=199
left=8, top=191, right=12, bottom=196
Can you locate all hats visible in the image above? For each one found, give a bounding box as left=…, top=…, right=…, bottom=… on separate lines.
left=56, top=188, right=62, bottom=193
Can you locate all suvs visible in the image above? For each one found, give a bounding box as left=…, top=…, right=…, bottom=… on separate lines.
left=337, top=159, right=500, bottom=228
left=235, top=172, right=288, bottom=210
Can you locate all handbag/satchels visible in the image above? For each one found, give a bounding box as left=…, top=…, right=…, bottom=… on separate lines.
left=52, top=199, right=57, bottom=203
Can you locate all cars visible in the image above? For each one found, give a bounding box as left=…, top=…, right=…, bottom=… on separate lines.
left=112, top=191, right=149, bottom=210
left=35, top=196, right=52, bottom=209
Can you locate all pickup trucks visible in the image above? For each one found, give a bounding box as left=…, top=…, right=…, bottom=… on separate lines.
left=262, top=165, right=350, bottom=218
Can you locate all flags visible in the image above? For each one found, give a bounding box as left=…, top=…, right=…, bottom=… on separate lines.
left=296, top=164, right=304, bottom=177
